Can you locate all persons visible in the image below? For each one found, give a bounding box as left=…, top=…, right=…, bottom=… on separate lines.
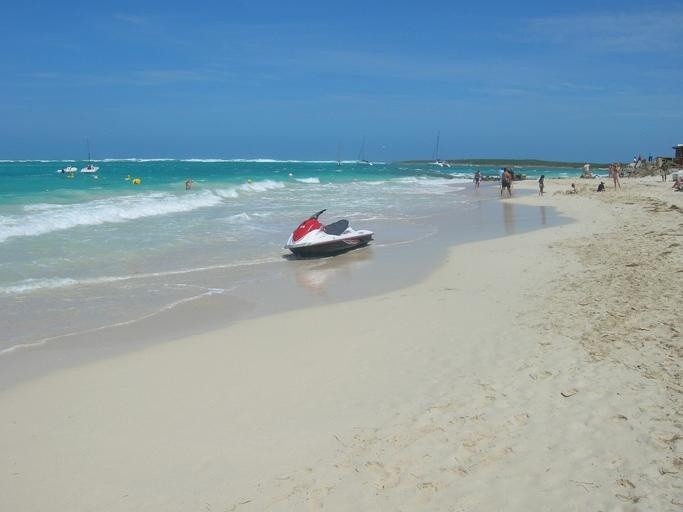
left=660, top=159, right=668, bottom=182
left=597, top=181, right=606, bottom=192
left=583, top=162, right=591, bottom=178
left=648, top=151, right=653, bottom=162
left=500, top=168, right=512, bottom=198
left=633, top=154, right=641, bottom=164
left=473, top=171, right=480, bottom=189
left=186, top=179, right=192, bottom=190
left=539, top=175, right=545, bottom=196
left=609, top=163, right=621, bottom=191
left=672, top=179, right=683, bottom=191
left=566, top=183, right=576, bottom=194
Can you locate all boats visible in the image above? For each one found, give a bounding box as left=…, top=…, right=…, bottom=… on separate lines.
left=429, top=132, right=450, bottom=167
left=58, top=166, right=78, bottom=173
left=81, top=165, right=100, bottom=173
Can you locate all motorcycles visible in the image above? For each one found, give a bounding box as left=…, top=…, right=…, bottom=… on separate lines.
left=285, top=209, right=374, bottom=256
